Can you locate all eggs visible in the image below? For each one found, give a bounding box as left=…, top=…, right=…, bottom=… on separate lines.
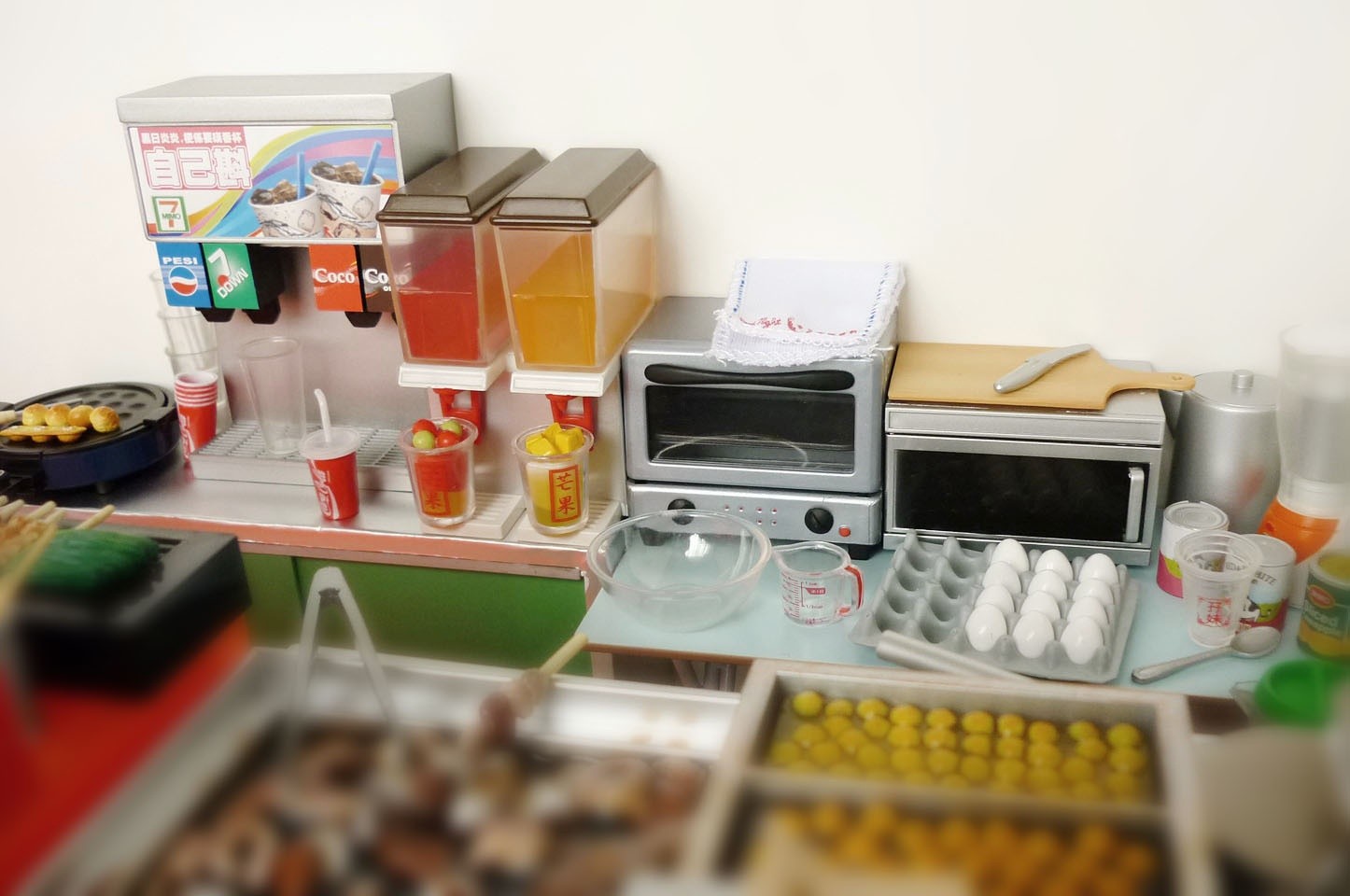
left=966, top=537, right=1118, bottom=662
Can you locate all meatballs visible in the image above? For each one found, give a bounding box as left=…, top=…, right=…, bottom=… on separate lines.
left=19, top=403, right=119, bottom=433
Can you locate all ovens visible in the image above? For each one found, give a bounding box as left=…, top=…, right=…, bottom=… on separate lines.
left=619, top=295, right=899, bottom=560
left=882, top=359, right=1165, bottom=568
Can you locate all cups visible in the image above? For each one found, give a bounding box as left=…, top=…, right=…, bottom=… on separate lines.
left=309, top=165, right=385, bottom=239
left=155, top=305, right=233, bottom=435
left=770, top=541, right=864, bottom=629
left=397, top=416, right=480, bottom=529
left=511, top=422, right=596, bottom=538
left=172, top=370, right=218, bottom=463
left=248, top=184, right=324, bottom=239
left=1156, top=500, right=1230, bottom=599
left=237, top=336, right=307, bottom=455
left=296, top=426, right=361, bottom=521
left=1172, top=529, right=1264, bottom=650
left=1164, top=368, right=1281, bottom=536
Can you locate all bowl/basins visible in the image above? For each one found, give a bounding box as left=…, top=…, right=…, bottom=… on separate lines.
left=586, top=508, right=773, bottom=633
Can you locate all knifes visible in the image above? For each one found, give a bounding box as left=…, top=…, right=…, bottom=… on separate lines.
left=994, top=343, right=1088, bottom=394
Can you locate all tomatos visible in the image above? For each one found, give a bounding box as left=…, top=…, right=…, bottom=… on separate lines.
left=410, top=420, right=460, bottom=452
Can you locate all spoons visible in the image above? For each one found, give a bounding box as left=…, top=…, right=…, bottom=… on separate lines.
left=1131, top=626, right=1281, bottom=684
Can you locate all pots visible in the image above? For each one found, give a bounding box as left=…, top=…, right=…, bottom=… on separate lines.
left=0, top=380, right=182, bottom=507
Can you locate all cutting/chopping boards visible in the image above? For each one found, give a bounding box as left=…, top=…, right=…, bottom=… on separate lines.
left=888, top=336, right=1196, bottom=410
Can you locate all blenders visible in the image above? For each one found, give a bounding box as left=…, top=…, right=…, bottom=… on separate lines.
left=1254, top=326, right=1350, bottom=610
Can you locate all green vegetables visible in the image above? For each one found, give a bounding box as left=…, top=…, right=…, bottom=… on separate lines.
left=22, top=529, right=161, bottom=590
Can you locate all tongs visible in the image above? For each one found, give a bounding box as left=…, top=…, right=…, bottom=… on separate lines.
left=282, top=563, right=402, bottom=752
